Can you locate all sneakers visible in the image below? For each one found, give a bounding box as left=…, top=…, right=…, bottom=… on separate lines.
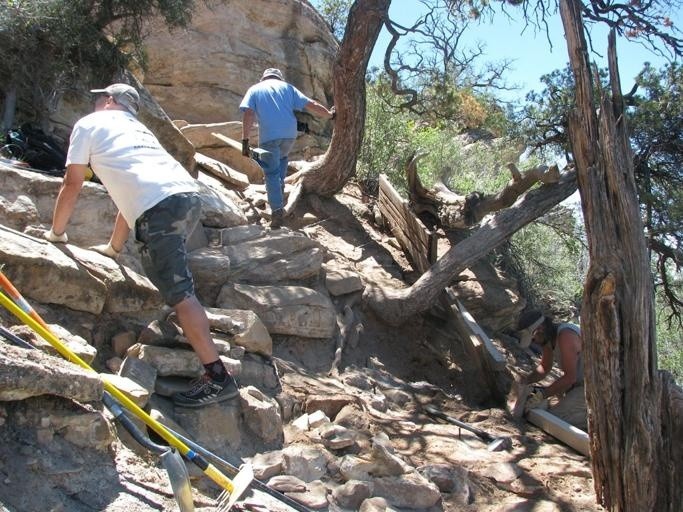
left=173, top=371, right=240, bottom=409
left=269, top=208, right=284, bottom=230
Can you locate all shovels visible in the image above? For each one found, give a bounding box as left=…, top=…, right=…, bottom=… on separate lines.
left=0, top=322, right=196, bottom=512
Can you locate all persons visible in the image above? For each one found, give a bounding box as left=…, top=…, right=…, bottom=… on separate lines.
left=514, top=309, right=581, bottom=414
left=43, top=83, right=240, bottom=409
left=238, top=67, right=337, bottom=227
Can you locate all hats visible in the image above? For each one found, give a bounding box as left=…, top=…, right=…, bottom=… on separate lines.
left=260, top=67, right=284, bottom=81
left=90, top=83, right=141, bottom=117
left=516, top=315, right=546, bottom=349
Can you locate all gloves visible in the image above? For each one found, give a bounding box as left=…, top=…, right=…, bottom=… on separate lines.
left=42, top=225, right=69, bottom=243
left=242, top=139, right=250, bottom=158
left=330, top=105, right=336, bottom=121
left=525, top=391, right=544, bottom=415
left=88, top=242, right=121, bottom=261
